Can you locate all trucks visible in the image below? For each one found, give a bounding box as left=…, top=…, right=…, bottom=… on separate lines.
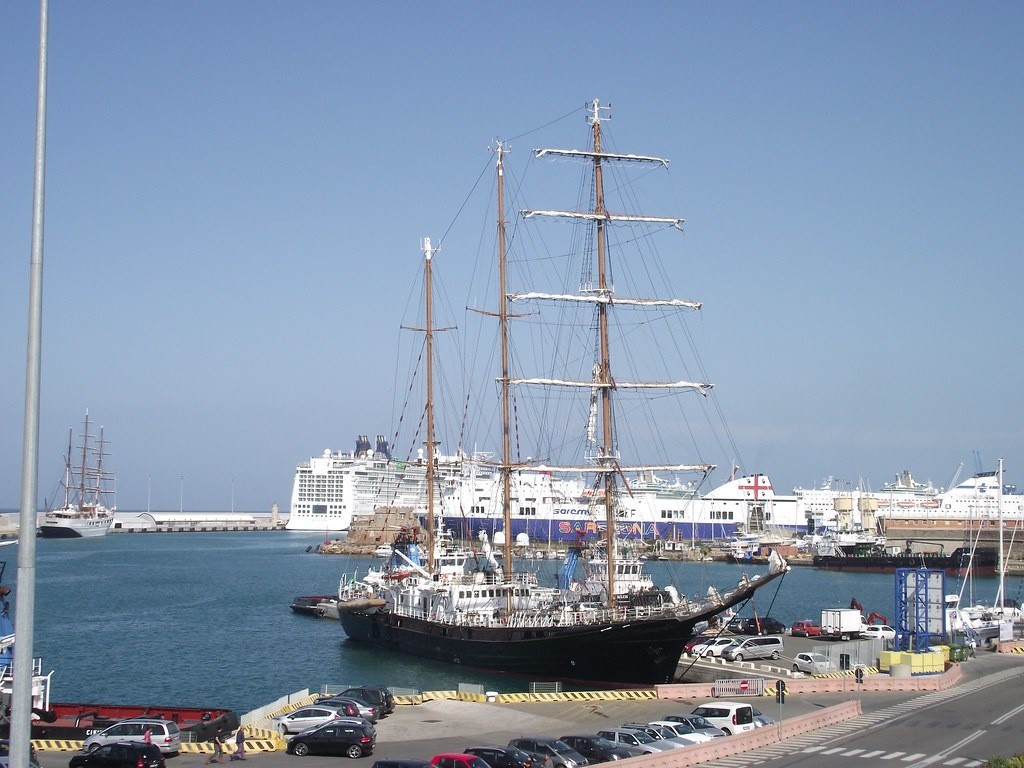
left=820, top=608, right=870, bottom=641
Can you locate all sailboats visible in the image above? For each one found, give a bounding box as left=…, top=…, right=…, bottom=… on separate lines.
left=948, top=497, right=1024, bottom=628
left=37, top=408, right=116, bottom=540
left=336, top=98, right=791, bottom=688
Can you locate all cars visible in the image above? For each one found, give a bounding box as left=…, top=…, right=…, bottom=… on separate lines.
left=864, top=623, right=896, bottom=641
left=372, top=711, right=730, bottom=768
left=685, top=635, right=734, bottom=658
left=272, top=685, right=397, bottom=759
left=752, top=703, right=776, bottom=727
left=81, top=718, right=183, bottom=754
left=951, top=627, right=981, bottom=648
left=0, top=738, right=45, bottom=768
left=728, top=616, right=786, bottom=635
left=791, top=619, right=822, bottom=637
left=793, top=653, right=837, bottom=675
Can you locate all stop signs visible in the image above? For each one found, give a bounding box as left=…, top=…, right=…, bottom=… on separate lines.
left=741, top=680, right=749, bottom=690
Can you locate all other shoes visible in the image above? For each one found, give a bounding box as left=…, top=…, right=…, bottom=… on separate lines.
left=206, top=757, right=210, bottom=763
left=218, top=757, right=224, bottom=764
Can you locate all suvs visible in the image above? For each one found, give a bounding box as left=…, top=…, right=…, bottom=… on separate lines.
left=69, top=741, right=169, bottom=768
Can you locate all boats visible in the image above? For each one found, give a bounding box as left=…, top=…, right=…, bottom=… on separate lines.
left=8, top=701, right=239, bottom=741
left=0, top=557, right=56, bottom=735
left=373, top=542, right=672, bottom=561
left=285, top=434, right=1024, bottom=535
left=568, top=538, right=655, bottom=594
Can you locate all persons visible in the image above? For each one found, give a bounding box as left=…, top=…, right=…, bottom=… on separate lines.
left=143, top=726, right=152, bottom=743
left=970, top=639, right=978, bottom=658
left=231, top=725, right=248, bottom=761
left=206, top=726, right=227, bottom=764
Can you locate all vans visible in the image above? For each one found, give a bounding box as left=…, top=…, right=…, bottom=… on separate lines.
left=721, top=636, right=784, bottom=662
left=691, top=701, right=755, bottom=735
